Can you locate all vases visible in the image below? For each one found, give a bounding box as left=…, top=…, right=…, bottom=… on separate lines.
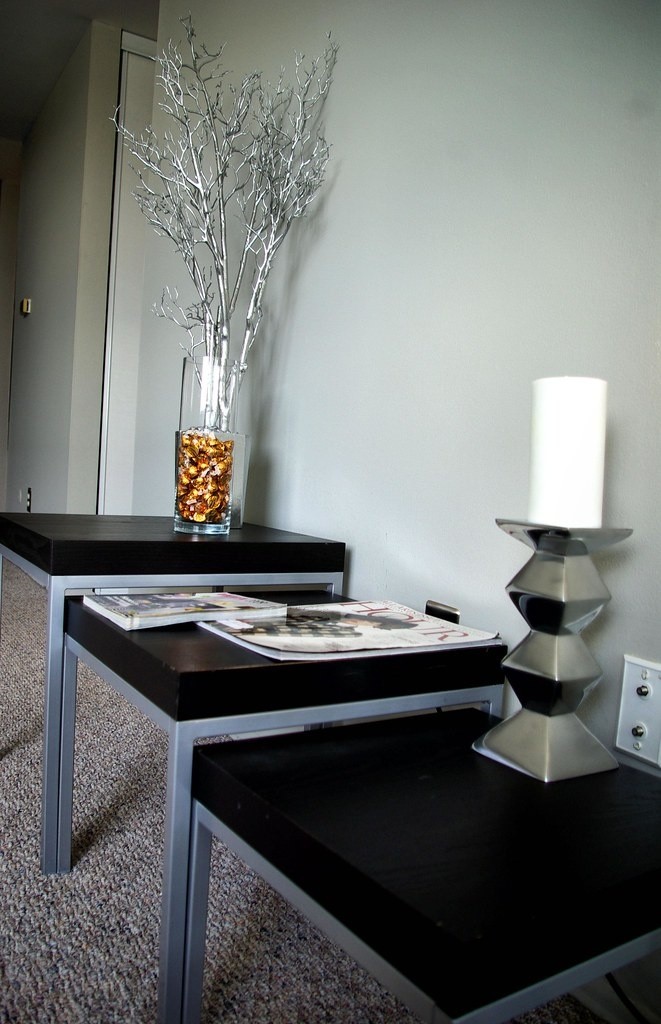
left=171, top=355, right=249, bottom=535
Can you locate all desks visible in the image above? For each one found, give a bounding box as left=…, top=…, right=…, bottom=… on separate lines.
left=182, top=706, right=661, bottom=1024
left=52, top=589, right=510, bottom=1024
left=1, top=509, right=351, bottom=874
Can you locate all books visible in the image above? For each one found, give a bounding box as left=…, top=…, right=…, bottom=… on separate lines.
left=82, top=591, right=288, bottom=632
left=195, top=600, right=503, bottom=662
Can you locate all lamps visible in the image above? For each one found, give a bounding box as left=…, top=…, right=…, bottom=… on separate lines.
left=466, top=377, right=633, bottom=789
